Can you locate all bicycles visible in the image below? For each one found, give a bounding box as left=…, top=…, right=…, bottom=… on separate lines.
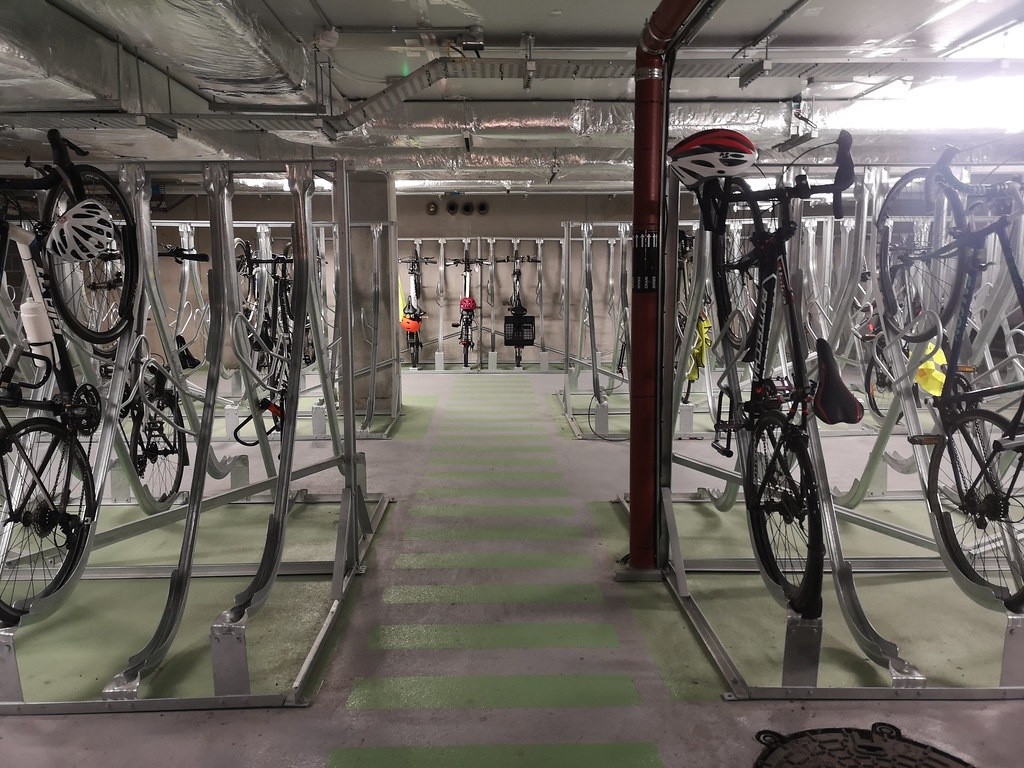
left=0, top=131, right=317, bottom=631
left=398, top=248, right=438, bottom=369
left=443, top=241, right=493, bottom=367
left=664, top=127, right=1023, bottom=619
left=494, top=244, right=542, bottom=367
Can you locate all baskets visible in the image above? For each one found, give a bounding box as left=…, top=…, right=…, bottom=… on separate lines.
left=504, top=315, right=535, bottom=346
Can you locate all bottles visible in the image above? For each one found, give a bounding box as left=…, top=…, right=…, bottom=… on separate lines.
left=20, top=297, right=60, bottom=368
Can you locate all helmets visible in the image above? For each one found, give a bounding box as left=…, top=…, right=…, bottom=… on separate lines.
left=45, top=199, right=113, bottom=262
left=460, top=298, right=476, bottom=309
left=401, top=317, right=422, bottom=332
left=667, top=129, right=758, bottom=188
